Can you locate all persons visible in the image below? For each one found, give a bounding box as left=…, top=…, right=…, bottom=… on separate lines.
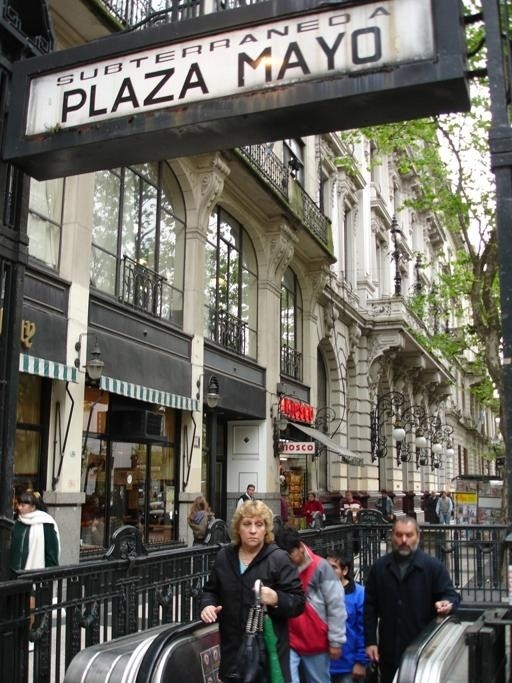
left=376, top=489, right=394, bottom=515
left=236, top=484, right=255, bottom=508
left=435, top=492, right=452, bottom=525
left=323, top=548, right=365, bottom=682
left=303, top=493, right=325, bottom=529
left=275, top=528, right=348, bottom=683
left=422, top=490, right=441, bottom=524
left=340, top=490, right=364, bottom=523
left=197, top=498, right=305, bottom=682
left=186, top=495, right=214, bottom=546
left=9, top=488, right=61, bottom=651
left=362, top=516, right=461, bottom=682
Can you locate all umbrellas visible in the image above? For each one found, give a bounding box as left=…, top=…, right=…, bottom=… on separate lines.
left=253, top=578, right=285, bottom=682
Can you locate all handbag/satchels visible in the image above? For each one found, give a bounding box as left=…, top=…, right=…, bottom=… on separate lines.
left=226, top=606, right=265, bottom=683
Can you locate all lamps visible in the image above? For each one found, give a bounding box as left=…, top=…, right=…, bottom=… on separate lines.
left=74, top=331, right=105, bottom=383
left=196, top=373, right=219, bottom=408
left=369, top=390, right=456, bottom=470
left=270, top=401, right=291, bottom=432
